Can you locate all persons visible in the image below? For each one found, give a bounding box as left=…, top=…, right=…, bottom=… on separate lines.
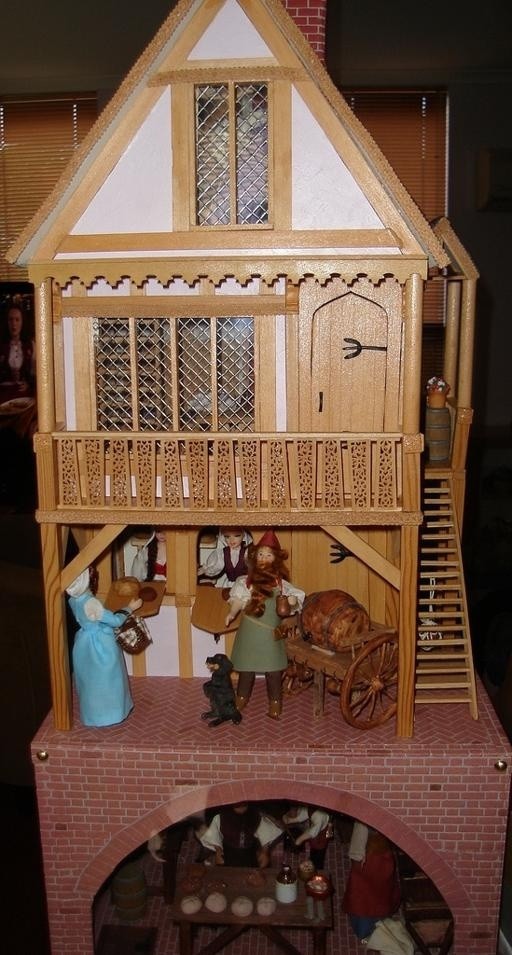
left=65, top=565, right=143, bottom=727
left=194, top=800, right=332, bottom=870
left=148, top=817, right=191, bottom=902
left=225, top=530, right=306, bottom=717
left=1, top=40, right=512, bottom=954
left=2, top=302, right=35, bottom=379
left=342, top=818, right=406, bottom=944
left=130, top=526, right=166, bottom=582
left=197, top=527, right=253, bottom=587
left=365, top=865, right=453, bottom=955
